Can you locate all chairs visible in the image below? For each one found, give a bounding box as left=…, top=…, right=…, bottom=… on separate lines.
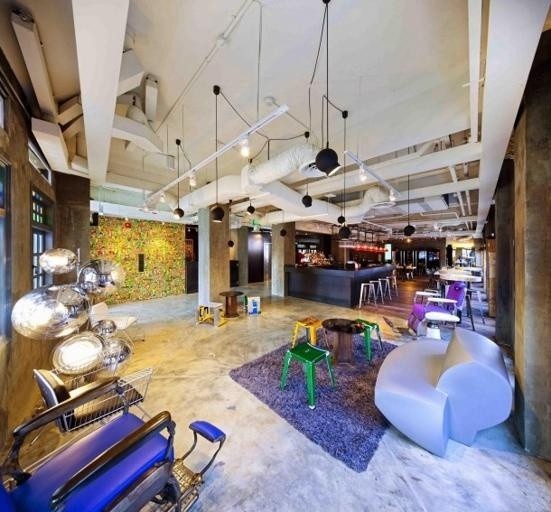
left=0, top=376, right=228, bottom=511
left=29, top=345, right=159, bottom=434
left=383, top=283, right=468, bottom=338
left=244, top=294, right=262, bottom=316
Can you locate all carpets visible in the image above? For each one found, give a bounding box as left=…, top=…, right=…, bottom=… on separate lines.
left=229, top=324, right=407, bottom=473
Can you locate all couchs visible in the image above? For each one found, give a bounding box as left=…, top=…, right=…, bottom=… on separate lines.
left=374, top=326, right=515, bottom=459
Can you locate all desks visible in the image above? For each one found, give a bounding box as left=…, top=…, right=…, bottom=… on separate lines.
left=219, top=291, right=244, bottom=318
left=322, top=319, right=367, bottom=365
left=413, top=264, right=485, bottom=326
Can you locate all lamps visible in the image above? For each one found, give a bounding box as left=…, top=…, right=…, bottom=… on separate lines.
left=279, top=210, right=287, bottom=237
left=210, top=84, right=225, bottom=224
left=311, top=1, right=340, bottom=177
left=188, top=168, right=197, bottom=186
left=172, top=139, right=185, bottom=220
left=246, top=158, right=256, bottom=216
left=403, top=147, right=415, bottom=238
left=240, top=136, right=251, bottom=158
left=301, top=132, right=313, bottom=209
left=337, top=172, right=345, bottom=224
left=227, top=209, right=236, bottom=249
left=338, top=111, right=352, bottom=240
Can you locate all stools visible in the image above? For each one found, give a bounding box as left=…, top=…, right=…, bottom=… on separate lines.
left=195, top=301, right=226, bottom=327
left=280, top=341, right=337, bottom=411
left=359, top=276, right=398, bottom=306
left=290, top=317, right=324, bottom=347
left=354, top=318, right=384, bottom=361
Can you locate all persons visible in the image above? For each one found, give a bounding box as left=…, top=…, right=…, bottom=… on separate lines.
left=405, top=261, right=416, bottom=280
